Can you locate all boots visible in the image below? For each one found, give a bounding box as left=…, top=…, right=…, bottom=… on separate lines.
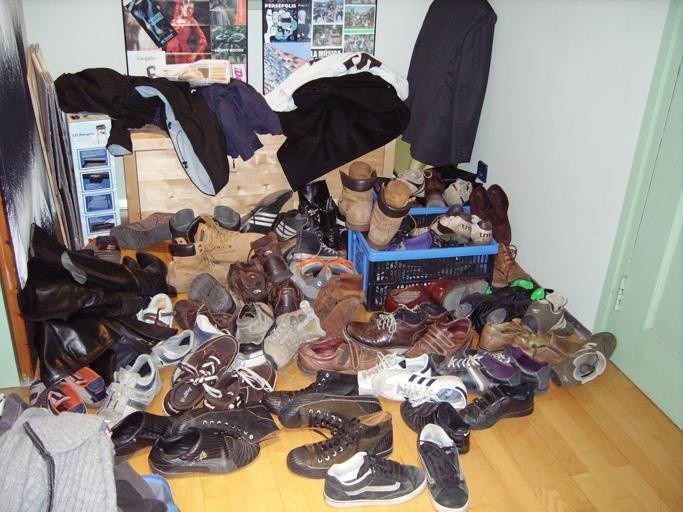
left=17, top=255, right=151, bottom=323
left=338, top=162, right=376, bottom=232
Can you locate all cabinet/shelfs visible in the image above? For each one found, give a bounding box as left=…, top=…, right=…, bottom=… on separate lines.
left=124, top=125, right=396, bottom=223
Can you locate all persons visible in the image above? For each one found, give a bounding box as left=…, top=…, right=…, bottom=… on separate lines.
left=166, top=0, right=209, bottom=63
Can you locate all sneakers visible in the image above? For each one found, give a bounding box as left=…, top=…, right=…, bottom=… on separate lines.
left=323, top=451, right=427, bottom=508
left=286, top=411, right=393, bottom=478
left=400, top=400, right=470, bottom=454
left=550, top=332, right=616, bottom=387
left=417, top=423, right=468, bottom=512
left=111, top=402, right=281, bottom=478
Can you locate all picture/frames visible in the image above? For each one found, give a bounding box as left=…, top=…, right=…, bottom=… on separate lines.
left=119, top=0, right=249, bottom=86
left=261, top=0, right=377, bottom=96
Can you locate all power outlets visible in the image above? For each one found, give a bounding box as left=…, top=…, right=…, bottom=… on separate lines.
left=476, top=160, right=488, bottom=183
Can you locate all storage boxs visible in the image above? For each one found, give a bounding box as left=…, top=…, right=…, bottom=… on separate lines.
left=67, top=113, right=122, bottom=247
